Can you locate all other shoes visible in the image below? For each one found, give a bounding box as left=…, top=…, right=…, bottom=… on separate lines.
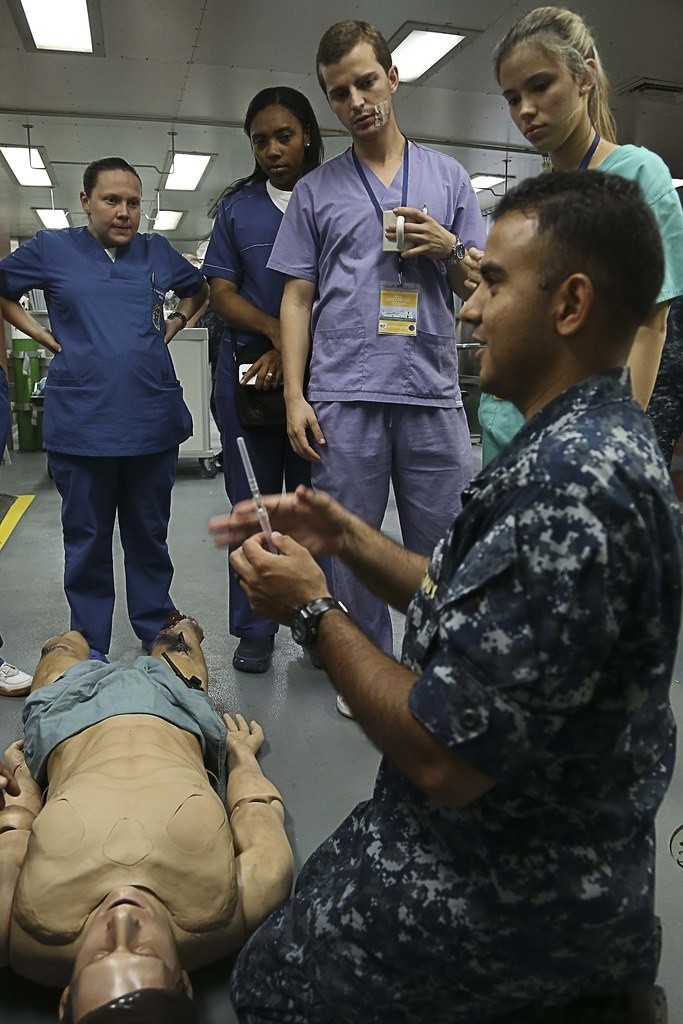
left=336, top=693, right=353, bottom=718
left=232, top=633, right=275, bottom=674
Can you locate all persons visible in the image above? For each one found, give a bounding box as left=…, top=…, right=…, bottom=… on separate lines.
left=0, top=7, right=683, bottom=1024
left=0, top=615, right=293, bottom=1024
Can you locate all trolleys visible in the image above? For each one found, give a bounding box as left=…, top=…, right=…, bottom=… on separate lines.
left=166, top=327, right=226, bottom=476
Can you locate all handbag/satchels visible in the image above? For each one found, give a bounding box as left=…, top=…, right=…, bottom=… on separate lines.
left=234, top=358, right=286, bottom=433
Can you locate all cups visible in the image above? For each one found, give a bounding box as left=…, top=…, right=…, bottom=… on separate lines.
left=382, top=209, right=418, bottom=252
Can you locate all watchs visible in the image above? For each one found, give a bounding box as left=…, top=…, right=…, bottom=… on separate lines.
left=291, top=597, right=350, bottom=645
left=440, top=234, right=464, bottom=267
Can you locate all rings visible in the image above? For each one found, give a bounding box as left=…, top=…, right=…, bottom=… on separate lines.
left=268, top=373, right=276, bottom=379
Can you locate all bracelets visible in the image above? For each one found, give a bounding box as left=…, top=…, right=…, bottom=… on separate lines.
left=167, top=311, right=188, bottom=330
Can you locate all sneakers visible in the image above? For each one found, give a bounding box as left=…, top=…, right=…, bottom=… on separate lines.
left=0, top=660, right=35, bottom=697
